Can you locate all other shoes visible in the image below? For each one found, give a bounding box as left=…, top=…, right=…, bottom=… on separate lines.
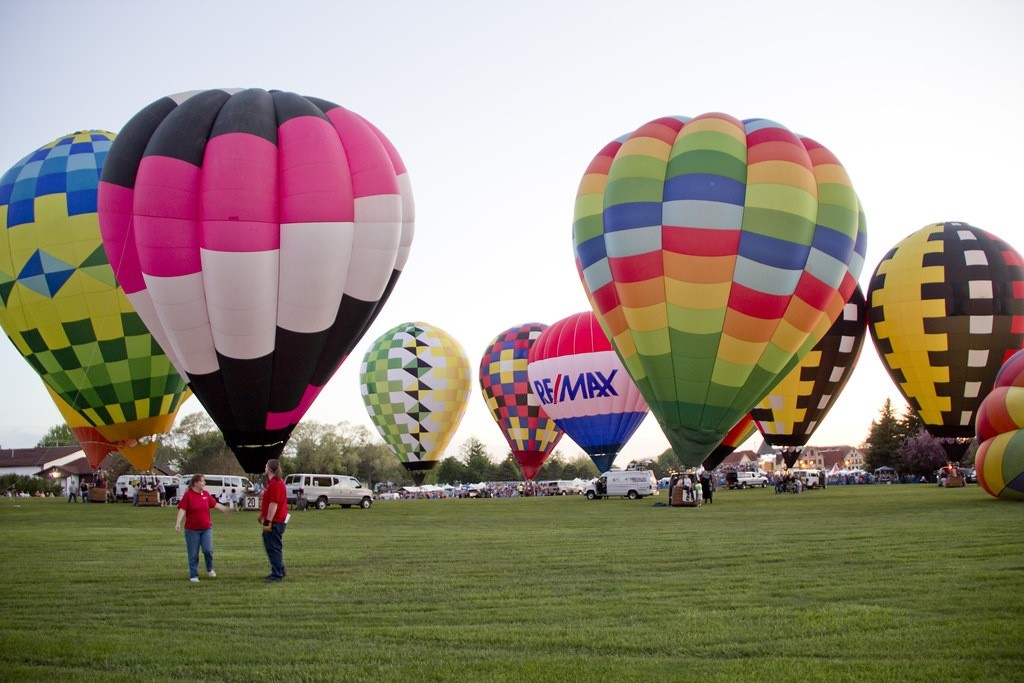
left=209, top=569, right=216, bottom=577
left=266, top=575, right=282, bottom=582
left=190, top=577, right=200, bottom=583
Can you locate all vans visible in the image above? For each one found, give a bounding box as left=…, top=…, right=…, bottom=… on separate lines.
left=176, top=475, right=255, bottom=502
left=548, top=481, right=581, bottom=496
left=726, top=470, right=770, bottom=489
left=115, top=475, right=181, bottom=500
left=582, top=471, right=659, bottom=500
left=284, top=474, right=375, bottom=509
left=794, top=471, right=821, bottom=489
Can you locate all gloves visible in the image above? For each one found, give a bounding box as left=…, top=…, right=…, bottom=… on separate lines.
left=262, top=519, right=271, bottom=532
left=257, top=514, right=263, bottom=524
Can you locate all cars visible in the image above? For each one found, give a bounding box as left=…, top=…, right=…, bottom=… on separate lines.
left=937, top=466, right=978, bottom=485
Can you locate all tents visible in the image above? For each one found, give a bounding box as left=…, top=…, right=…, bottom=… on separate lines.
left=442, top=483, right=454, bottom=489
left=875, top=466, right=896, bottom=474
left=420, top=485, right=443, bottom=492
left=396, top=487, right=421, bottom=493
left=828, top=464, right=863, bottom=476
left=456, top=482, right=487, bottom=491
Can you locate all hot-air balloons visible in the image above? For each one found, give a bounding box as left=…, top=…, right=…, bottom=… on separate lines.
left=974, top=343, right=1024, bottom=499
left=480, top=310, right=650, bottom=497
left=866, top=220, right=1023, bottom=487
left=0, top=126, right=196, bottom=485
left=99, top=86, right=416, bottom=498
left=358, top=321, right=473, bottom=490
left=573, top=112, right=870, bottom=506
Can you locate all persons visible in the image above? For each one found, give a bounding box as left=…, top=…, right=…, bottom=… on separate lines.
left=238, top=479, right=264, bottom=511
left=217, top=489, right=238, bottom=512
left=695, top=480, right=702, bottom=507
left=941, top=468, right=967, bottom=487
left=96, top=476, right=107, bottom=489
left=3, top=488, right=53, bottom=497
left=175, top=473, right=229, bottom=582
left=68, top=481, right=77, bottom=503
left=878, top=472, right=921, bottom=483
left=684, top=474, right=691, bottom=500
left=257, top=459, right=289, bottom=583
left=774, top=475, right=803, bottom=494
left=80, top=479, right=90, bottom=503
left=132, top=479, right=166, bottom=507
left=487, top=482, right=549, bottom=498
left=405, top=492, right=466, bottom=499
